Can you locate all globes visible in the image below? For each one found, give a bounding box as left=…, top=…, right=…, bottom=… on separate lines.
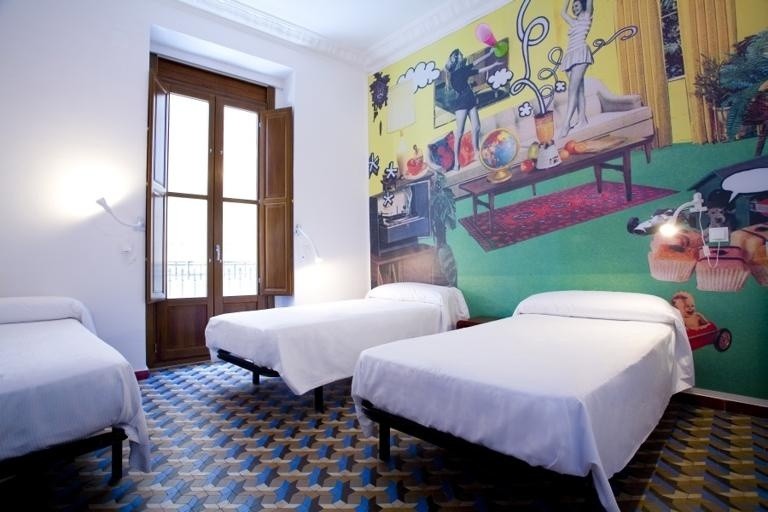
left=479, top=128, right=520, bottom=183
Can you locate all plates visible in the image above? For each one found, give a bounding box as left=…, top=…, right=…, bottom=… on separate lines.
left=404, top=166, right=430, bottom=180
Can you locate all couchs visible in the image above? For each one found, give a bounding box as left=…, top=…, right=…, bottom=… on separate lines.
left=427, top=77, right=655, bottom=198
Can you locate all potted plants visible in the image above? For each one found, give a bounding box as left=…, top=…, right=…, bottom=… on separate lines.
left=426, top=174, right=459, bottom=291
left=689, top=52, right=754, bottom=139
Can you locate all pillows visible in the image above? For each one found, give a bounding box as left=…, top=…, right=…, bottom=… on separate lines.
left=428, top=131, right=477, bottom=170
left=2, top=296, right=75, bottom=327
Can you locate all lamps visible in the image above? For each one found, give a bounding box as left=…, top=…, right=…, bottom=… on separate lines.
left=386, top=77, right=416, bottom=178
left=96, top=197, right=147, bottom=235
left=659, top=191, right=708, bottom=238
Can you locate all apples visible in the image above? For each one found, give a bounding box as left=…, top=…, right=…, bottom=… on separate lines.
left=520, top=160, right=535, bottom=173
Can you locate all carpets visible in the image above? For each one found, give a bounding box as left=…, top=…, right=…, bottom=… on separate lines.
left=459, top=180, right=682, bottom=253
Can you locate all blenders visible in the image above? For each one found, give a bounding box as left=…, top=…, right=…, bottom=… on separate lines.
left=534, top=110, right=562, bottom=169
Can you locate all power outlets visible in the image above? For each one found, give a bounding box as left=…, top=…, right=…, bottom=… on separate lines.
left=709, top=227, right=730, bottom=243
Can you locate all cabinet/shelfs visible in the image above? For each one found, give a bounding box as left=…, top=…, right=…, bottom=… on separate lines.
left=371, top=243, right=448, bottom=288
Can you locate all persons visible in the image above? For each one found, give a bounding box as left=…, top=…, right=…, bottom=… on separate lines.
left=443, top=47, right=503, bottom=171
left=412, top=144, right=422, bottom=160
left=672, top=291, right=711, bottom=329
left=559, top=1, right=594, bottom=139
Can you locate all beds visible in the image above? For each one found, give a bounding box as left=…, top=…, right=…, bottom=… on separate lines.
left=356, top=290, right=695, bottom=512
left=205, top=283, right=442, bottom=418
left=1, top=295, right=145, bottom=482
